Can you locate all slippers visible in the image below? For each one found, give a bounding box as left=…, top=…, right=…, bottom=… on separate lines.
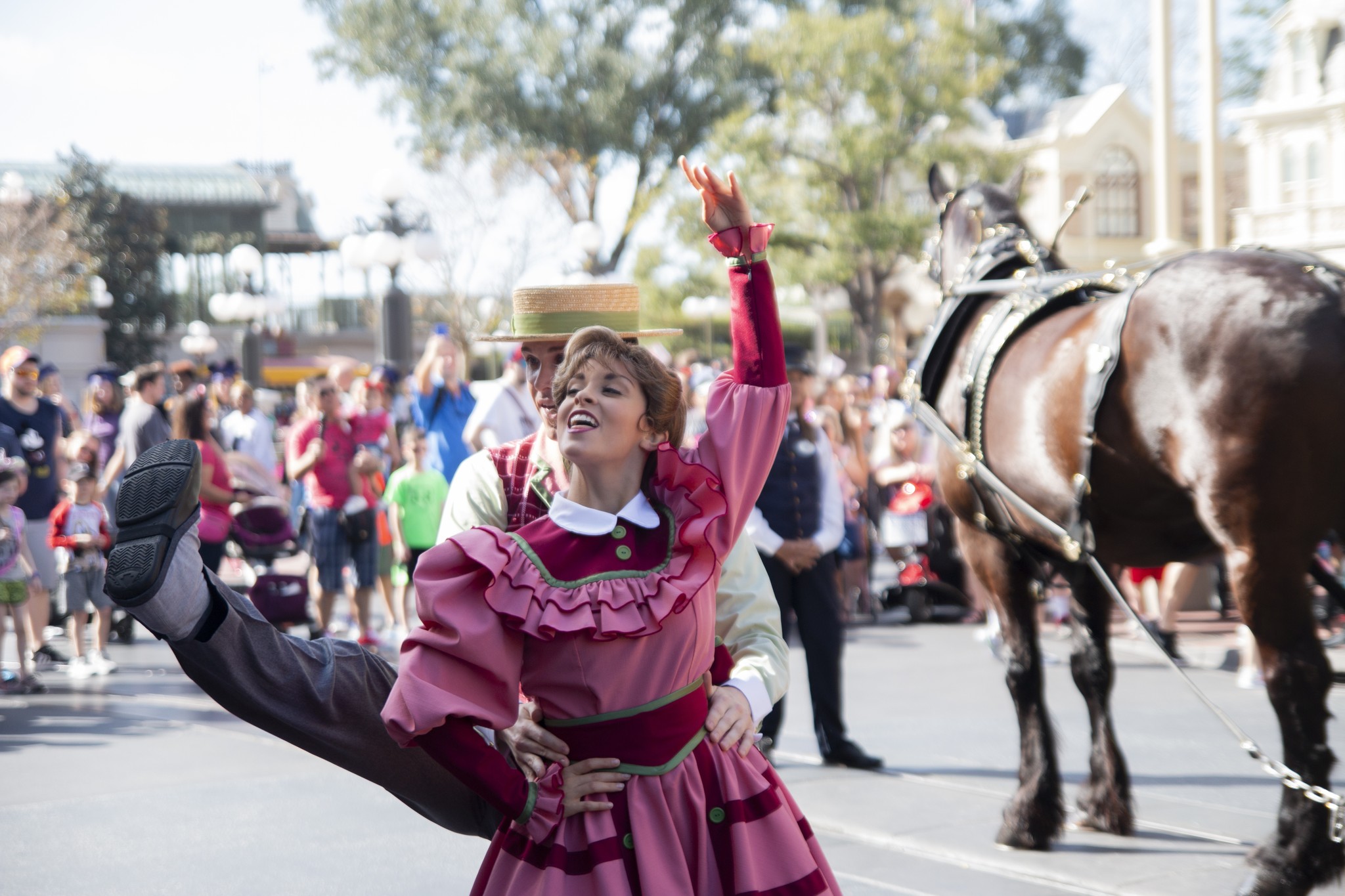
left=105, top=439, right=206, bottom=605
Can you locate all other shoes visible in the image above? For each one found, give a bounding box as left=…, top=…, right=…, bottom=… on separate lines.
left=90, top=651, right=119, bottom=674
left=960, top=608, right=985, bottom=624
left=72, top=657, right=98, bottom=679
left=822, top=742, right=882, bottom=770
left=19, top=676, right=44, bottom=693
left=0, top=669, right=24, bottom=695
left=1147, top=620, right=1182, bottom=664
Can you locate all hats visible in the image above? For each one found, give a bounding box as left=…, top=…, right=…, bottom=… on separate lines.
left=784, top=346, right=817, bottom=375
left=473, top=282, right=684, bottom=342
left=66, top=463, right=95, bottom=483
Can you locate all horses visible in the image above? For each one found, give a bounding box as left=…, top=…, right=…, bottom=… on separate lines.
left=927, top=161, right=1345, bottom=896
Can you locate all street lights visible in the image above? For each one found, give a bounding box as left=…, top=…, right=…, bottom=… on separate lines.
left=356, top=198, right=433, bottom=378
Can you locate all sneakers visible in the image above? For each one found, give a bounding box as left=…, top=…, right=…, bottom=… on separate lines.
left=31, top=645, right=69, bottom=671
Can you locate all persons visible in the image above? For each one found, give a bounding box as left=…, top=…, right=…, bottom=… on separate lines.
left=0, top=345, right=277, bottom=694
left=1126, top=561, right=1198, bottom=661
left=803, top=360, right=987, bottom=623
left=283, top=323, right=542, bottom=652
left=744, top=344, right=883, bottom=770
left=100, top=283, right=791, bottom=841
left=381, top=156, right=842, bottom=896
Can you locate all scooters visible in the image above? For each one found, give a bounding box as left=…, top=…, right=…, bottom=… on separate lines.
left=872, top=458, right=974, bottom=621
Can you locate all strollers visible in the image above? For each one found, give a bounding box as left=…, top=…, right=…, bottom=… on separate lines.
left=222, top=492, right=319, bottom=638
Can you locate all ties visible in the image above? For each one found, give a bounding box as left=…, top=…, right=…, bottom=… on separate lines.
left=787, top=419, right=799, bottom=436
